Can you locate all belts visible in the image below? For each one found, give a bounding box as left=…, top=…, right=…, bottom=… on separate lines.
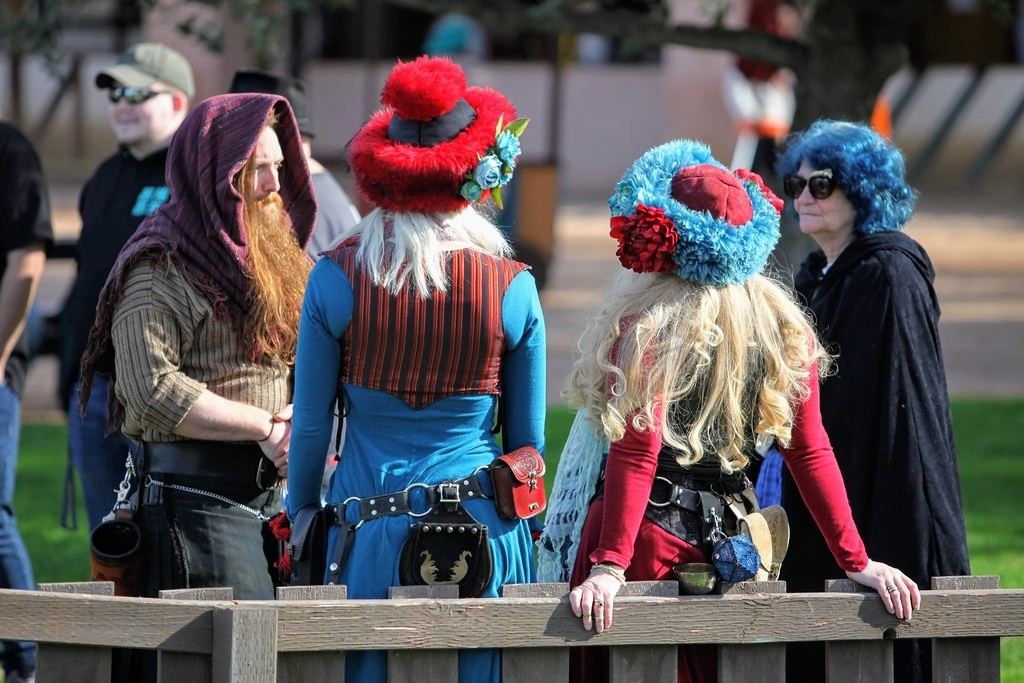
left=147, top=437, right=287, bottom=491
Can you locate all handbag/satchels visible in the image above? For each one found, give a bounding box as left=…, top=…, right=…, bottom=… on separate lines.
left=286, top=503, right=329, bottom=584
left=491, top=448, right=547, bottom=522
left=398, top=499, right=495, bottom=599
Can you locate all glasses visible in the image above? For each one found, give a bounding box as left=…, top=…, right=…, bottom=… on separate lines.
left=781, top=172, right=839, bottom=199
left=107, top=85, right=168, bottom=104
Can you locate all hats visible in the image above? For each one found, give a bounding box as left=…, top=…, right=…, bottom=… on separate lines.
left=228, top=69, right=316, bottom=137
left=96, top=41, right=196, bottom=104
left=607, top=138, right=785, bottom=289
left=347, top=55, right=531, bottom=214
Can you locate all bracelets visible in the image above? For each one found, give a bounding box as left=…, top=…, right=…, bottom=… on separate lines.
left=589, top=564, right=626, bottom=584
left=256, top=411, right=275, bottom=442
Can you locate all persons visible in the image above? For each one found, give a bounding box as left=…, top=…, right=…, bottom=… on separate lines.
left=284, top=53, right=546, bottom=683
left=0, top=119, right=38, bottom=682
left=77, top=95, right=318, bottom=601
left=57, top=42, right=194, bottom=582
left=229, top=67, right=362, bottom=261
left=534, top=135, right=922, bottom=683
left=774, top=118, right=971, bottom=683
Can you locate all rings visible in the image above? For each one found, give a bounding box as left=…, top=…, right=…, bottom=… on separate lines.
left=583, top=613, right=592, bottom=618
left=888, top=584, right=899, bottom=593
left=574, top=586, right=581, bottom=595
left=596, top=616, right=604, bottom=622
left=594, top=599, right=604, bottom=606
